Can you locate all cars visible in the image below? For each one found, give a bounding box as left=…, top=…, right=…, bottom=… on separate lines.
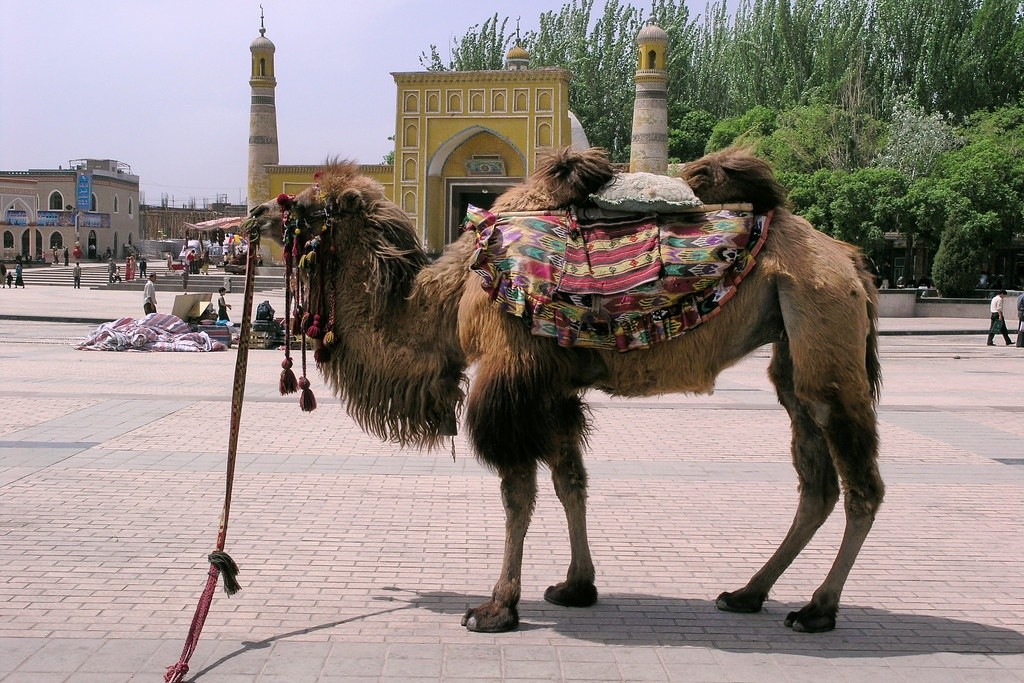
left=918, top=285, right=940, bottom=297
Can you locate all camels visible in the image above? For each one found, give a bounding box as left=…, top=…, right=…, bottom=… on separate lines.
left=242, top=127, right=888, bottom=633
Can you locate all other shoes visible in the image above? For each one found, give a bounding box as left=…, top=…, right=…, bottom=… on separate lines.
left=1006, top=342, right=1014, bottom=345
left=23, top=287, right=24, bottom=288
left=987, top=344, right=996, bottom=346
left=15, top=287, right=17, bottom=288
left=78, top=287, right=80, bottom=289
left=202, top=272, right=204, bottom=274
left=206, top=274, right=208, bottom=275
left=3, top=287, right=5, bottom=288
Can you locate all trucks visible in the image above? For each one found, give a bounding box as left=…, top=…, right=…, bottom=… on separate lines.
left=177, top=240, right=224, bottom=265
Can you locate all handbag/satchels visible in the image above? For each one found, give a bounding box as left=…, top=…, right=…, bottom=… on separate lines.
left=256, top=301, right=275, bottom=321
left=1019, top=321, right=1024, bottom=332
left=991, top=319, right=1003, bottom=333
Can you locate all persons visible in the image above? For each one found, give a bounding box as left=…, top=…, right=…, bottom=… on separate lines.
left=0, top=261, right=25, bottom=288
left=986, top=290, right=1016, bottom=346
left=1016, top=294, right=1024, bottom=348
left=217, top=287, right=232, bottom=321
left=72, top=262, right=82, bottom=290
left=107, top=256, right=148, bottom=284
left=143, top=272, right=157, bottom=315
left=201, top=251, right=210, bottom=275
left=17, top=242, right=113, bottom=269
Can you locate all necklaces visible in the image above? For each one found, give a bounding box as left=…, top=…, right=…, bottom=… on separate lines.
left=188, top=250, right=196, bottom=274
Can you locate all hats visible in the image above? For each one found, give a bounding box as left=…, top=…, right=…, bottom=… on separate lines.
left=183, top=266, right=189, bottom=270
